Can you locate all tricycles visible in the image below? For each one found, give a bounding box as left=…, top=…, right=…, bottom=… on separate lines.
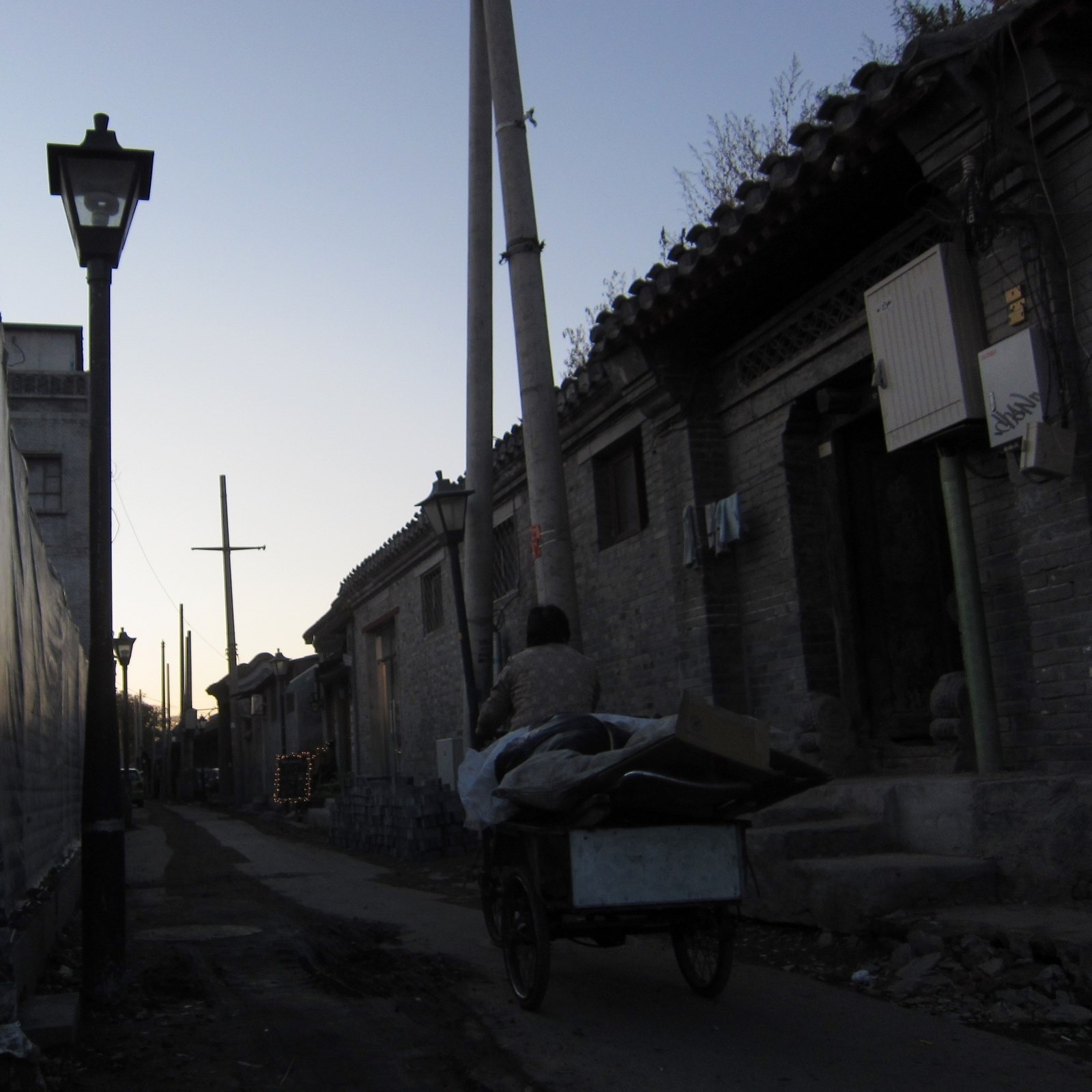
left=477, top=783, right=756, bottom=1007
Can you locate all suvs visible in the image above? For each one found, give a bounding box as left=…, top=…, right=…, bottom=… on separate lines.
left=119, top=768, right=147, bottom=805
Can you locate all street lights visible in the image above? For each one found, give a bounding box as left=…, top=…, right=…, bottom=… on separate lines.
left=45, top=116, right=160, bottom=1024
left=111, top=625, right=137, bottom=788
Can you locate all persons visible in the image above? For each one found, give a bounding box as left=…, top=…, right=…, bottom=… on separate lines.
left=475, top=602, right=607, bottom=931
